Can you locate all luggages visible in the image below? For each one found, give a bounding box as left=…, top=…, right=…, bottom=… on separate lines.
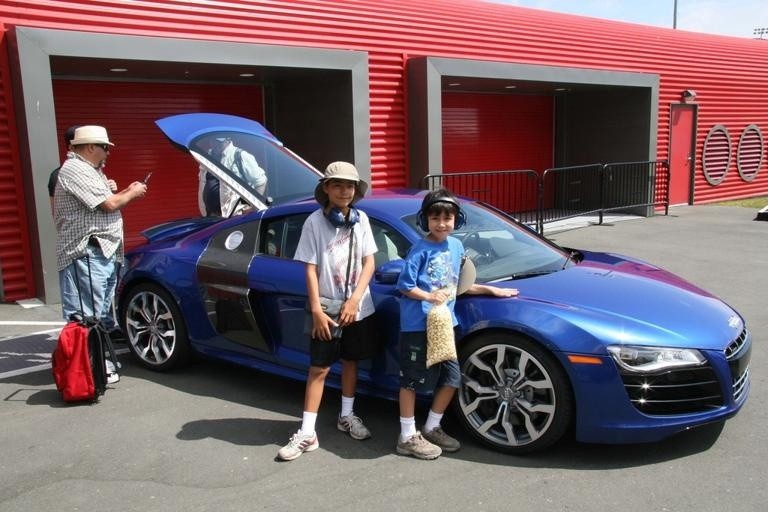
left=52, top=254, right=120, bottom=404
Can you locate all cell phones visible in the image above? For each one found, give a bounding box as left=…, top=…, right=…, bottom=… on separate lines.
left=142, top=171, right=153, bottom=183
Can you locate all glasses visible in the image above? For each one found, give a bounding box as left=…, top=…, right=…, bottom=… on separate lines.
left=94, top=144, right=108, bottom=152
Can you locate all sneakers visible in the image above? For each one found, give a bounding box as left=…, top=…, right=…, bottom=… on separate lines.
left=422, top=426, right=461, bottom=452
left=277, top=429, right=320, bottom=461
left=337, top=412, right=371, bottom=440
left=104, top=359, right=122, bottom=383
left=397, top=430, right=442, bottom=460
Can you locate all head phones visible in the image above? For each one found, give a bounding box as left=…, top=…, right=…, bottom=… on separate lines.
left=322, top=205, right=359, bottom=226
left=416, top=197, right=468, bottom=232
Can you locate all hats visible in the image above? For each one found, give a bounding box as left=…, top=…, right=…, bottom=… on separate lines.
left=314, top=161, right=367, bottom=206
left=70, top=125, right=115, bottom=146
left=457, top=256, right=477, bottom=297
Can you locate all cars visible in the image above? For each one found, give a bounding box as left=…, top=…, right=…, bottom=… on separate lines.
left=116, top=111, right=751, bottom=459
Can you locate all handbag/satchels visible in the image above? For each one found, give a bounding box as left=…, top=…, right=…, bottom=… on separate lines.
left=303, top=296, right=346, bottom=339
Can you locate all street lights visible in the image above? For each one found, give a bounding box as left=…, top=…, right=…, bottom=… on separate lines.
left=753, top=28, right=768, bottom=39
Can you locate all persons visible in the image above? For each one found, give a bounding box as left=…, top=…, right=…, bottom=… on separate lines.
left=47, top=125, right=117, bottom=219
left=275, top=161, right=379, bottom=462
left=396, top=188, right=519, bottom=460
left=54, top=126, right=147, bottom=383
left=197, top=131, right=268, bottom=218
